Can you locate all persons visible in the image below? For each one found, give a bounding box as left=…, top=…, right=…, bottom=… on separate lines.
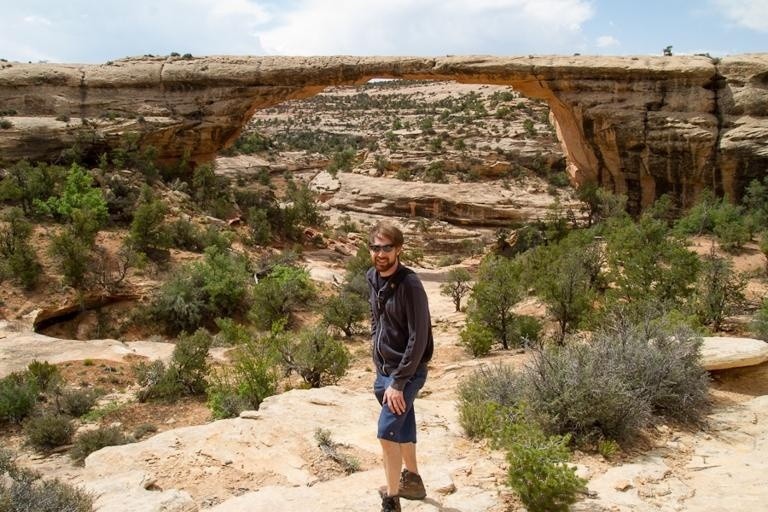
left=365, top=223, right=434, bottom=512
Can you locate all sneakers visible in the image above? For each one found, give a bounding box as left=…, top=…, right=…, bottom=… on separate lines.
left=379, top=468, right=426, bottom=500
left=380, top=496, right=401, bottom=511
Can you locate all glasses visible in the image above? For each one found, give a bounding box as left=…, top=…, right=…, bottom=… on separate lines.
left=370, top=243, right=396, bottom=252
